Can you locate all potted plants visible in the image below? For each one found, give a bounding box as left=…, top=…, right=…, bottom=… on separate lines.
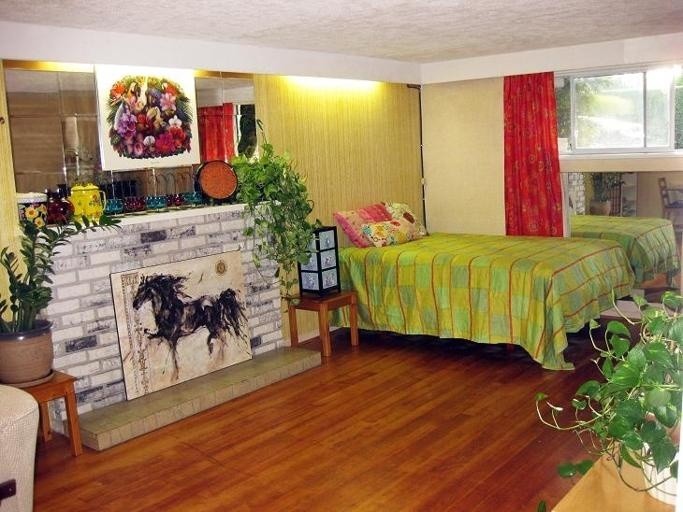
left=581, top=171, right=635, bottom=217
left=531, top=286, right=683, bottom=509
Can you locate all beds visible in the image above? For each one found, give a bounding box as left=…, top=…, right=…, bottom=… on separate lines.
left=335, top=232, right=631, bottom=360
left=569, top=214, right=678, bottom=289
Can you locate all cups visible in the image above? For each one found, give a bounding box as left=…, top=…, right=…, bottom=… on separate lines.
left=106, top=192, right=204, bottom=214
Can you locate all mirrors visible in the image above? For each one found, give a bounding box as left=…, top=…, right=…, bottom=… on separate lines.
left=2, top=56, right=265, bottom=228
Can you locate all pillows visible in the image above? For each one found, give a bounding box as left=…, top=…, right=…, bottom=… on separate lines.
left=334, top=199, right=430, bottom=250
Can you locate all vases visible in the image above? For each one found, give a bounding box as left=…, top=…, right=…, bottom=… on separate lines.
left=1, top=316, right=57, bottom=389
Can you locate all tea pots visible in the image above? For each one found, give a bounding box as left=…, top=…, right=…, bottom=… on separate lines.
left=66, top=184, right=84, bottom=216
left=77, top=183, right=105, bottom=221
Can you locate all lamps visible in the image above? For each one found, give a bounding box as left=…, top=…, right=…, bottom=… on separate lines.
left=294, top=224, right=339, bottom=296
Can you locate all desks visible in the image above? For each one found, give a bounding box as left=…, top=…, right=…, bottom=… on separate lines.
left=26, top=371, right=85, bottom=460
left=288, top=290, right=360, bottom=358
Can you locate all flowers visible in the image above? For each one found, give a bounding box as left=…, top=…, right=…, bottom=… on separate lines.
left=0, top=200, right=124, bottom=337
left=109, top=74, right=194, bottom=161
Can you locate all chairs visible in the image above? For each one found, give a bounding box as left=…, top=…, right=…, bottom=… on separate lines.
left=658, top=175, right=682, bottom=225
